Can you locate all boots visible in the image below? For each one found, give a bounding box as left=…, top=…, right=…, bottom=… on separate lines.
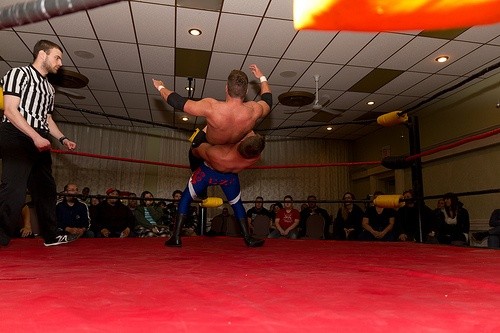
left=165, top=213, right=188, bottom=247
left=238, top=218, right=265, bottom=247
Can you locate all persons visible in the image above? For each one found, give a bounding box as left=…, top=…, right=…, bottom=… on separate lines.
left=152, top=64, right=273, bottom=171
left=247, top=189, right=470, bottom=247
left=165, top=125, right=266, bottom=247
left=16, top=205, right=34, bottom=237
left=472, top=209, right=500, bottom=249
left=0, top=39, right=81, bottom=246
left=56, top=183, right=197, bottom=238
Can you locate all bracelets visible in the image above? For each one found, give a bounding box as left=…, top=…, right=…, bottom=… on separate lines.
left=60, top=136, right=69, bottom=144
left=157, top=85, right=165, bottom=91
left=260, top=76, right=268, bottom=82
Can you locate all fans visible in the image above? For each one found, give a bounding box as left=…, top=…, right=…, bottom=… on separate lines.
left=49, top=83, right=86, bottom=99
left=284, top=74, right=343, bottom=116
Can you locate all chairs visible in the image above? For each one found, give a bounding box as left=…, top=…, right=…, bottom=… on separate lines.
left=252, top=215, right=270, bottom=239
left=300, top=215, right=325, bottom=240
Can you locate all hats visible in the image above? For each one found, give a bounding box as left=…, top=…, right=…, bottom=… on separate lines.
left=106, top=187, right=121, bottom=195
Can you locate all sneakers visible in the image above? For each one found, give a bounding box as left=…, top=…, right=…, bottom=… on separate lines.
left=0, top=234, right=9, bottom=246
left=43, top=227, right=80, bottom=246
